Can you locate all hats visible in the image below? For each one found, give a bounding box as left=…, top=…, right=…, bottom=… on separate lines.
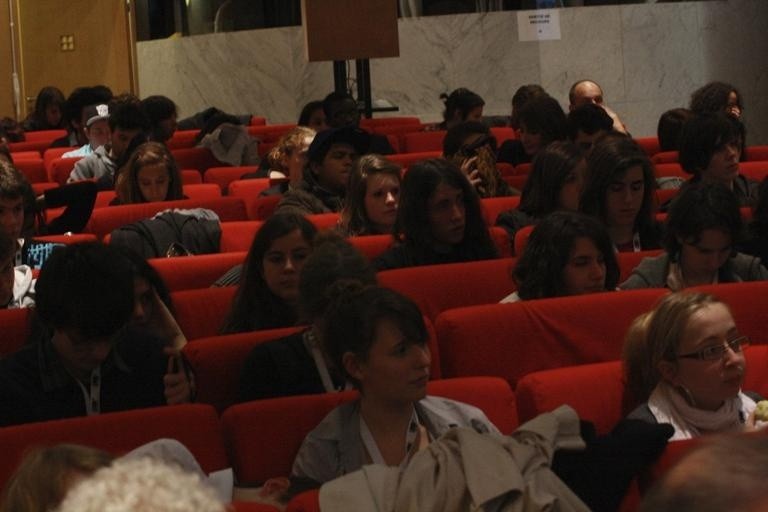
left=82, top=104, right=113, bottom=128
left=306, top=124, right=372, bottom=161
left=65, top=86, right=113, bottom=119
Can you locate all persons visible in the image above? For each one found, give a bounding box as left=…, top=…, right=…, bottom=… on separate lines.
left=580, top=140, right=675, bottom=251
left=277, top=127, right=373, bottom=218
left=109, top=141, right=191, bottom=207
left=297, top=100, right=330, bottom=133
left=565, top=79, right=629, bottom=135
left=259, top=127, right=316, bottom=195
left=19, top=86, right=69, bottom=133
left=238, top=235, right=382, bottom=400
left=617, top=290, right=768, bottom=492
left=117, top=244, right=189, bottom=382
left=62, top=103, right=113, bottom=159
left=323, top=93, right=361, bottom=129
left=423, top=88, right=485, bottom=131
left=335, top=153, right=407, bottom=280
left=0, top=240, right=196, bottom=427
left=497, top=82, right=544, bottom=130
left=499, top=208, right=622, bottom=303
left=67, top=96, right=152, bottom=191
left=497, top=138, right=589, bottom=257
left=0, top=240, right=39, bottom=311
left=660, top=111, right=764, bottom=212
left=497, top=97, right=566, bottom=166
left=441, top=123, right=526, bottom=196
left=656, top=104, right=695, bottom=152
left=688, top=79, right=745, bottom=120
left=0, top=157, right=99, bottom=244
left=260, top=286, right=502, bottom=502
left=638, top=434, right=768, bottom=512
left=621, top=172, right=768, bottom=289
left=217, top=211, right=322, bottom=335
left=118, top=95, right=179, bottom=168
left=373, top=158, right=502, bottom=270
left=566, top=105, right=614, bottom=149
left=0, top=444, right=113, bottom=512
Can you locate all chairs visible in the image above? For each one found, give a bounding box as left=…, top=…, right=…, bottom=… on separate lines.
left=0, top=109, right=765, bottom=511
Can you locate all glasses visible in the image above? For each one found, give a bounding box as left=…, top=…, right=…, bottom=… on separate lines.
left=685, top=240, right=734, bottom=254
left=677, top=335, right=753, bottom=361
